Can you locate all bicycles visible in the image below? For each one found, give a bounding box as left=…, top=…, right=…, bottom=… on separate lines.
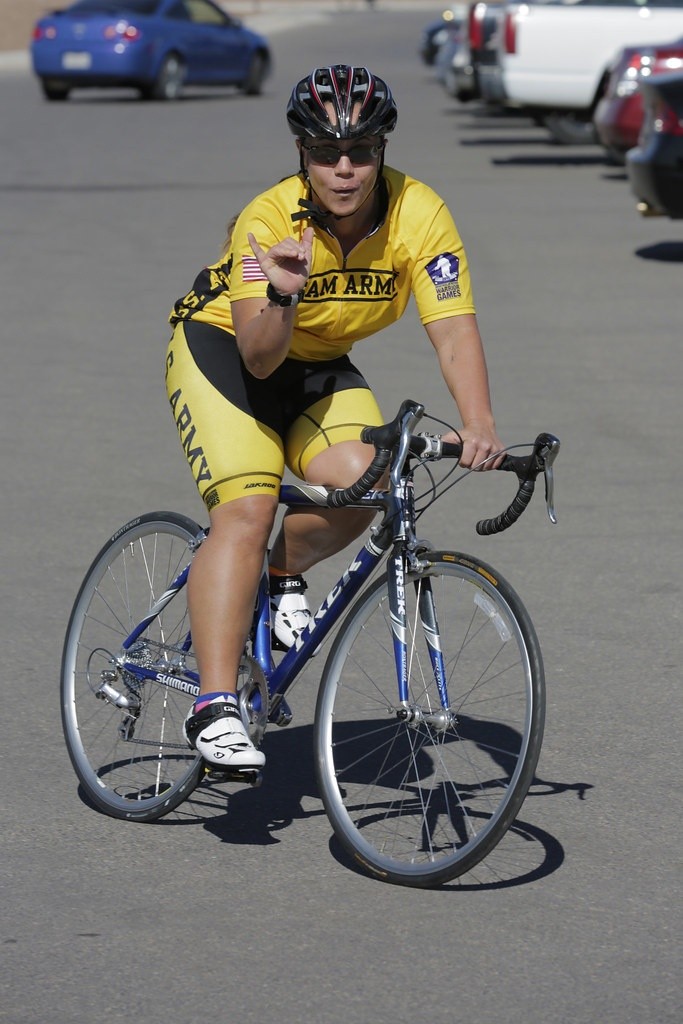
left=58, top=399, right=559, bottom=888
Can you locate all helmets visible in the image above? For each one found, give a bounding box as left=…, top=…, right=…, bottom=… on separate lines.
left=286, top=64, right=398, bottom=140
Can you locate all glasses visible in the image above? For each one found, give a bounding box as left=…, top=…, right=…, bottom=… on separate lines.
left=301, top=143, right=383, bottom=164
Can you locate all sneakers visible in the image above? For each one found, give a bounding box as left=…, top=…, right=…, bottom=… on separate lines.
left=270, top=573, right=321, bottom=657
left=182, top=694, right=266, bottom=770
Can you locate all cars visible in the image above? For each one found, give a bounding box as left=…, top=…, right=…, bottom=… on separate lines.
left=592, top=37, right=683, bottom=166
left=436, top=28, right=481, bottom=103
left=496, top=0, right=683, bottom=140
left=467, top=0, right=683, bottom=108
left=419, top=16, right=469, bottom=64
left=30, top=0, right=272, bottom=100
left=624, top=72, right=683, bottom=222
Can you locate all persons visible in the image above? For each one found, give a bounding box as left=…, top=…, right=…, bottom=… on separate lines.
left=161, top=63, right=505, bottom=764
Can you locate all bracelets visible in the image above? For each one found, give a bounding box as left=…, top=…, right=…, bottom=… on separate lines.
left=266, top=281, right=305, bottom=304
